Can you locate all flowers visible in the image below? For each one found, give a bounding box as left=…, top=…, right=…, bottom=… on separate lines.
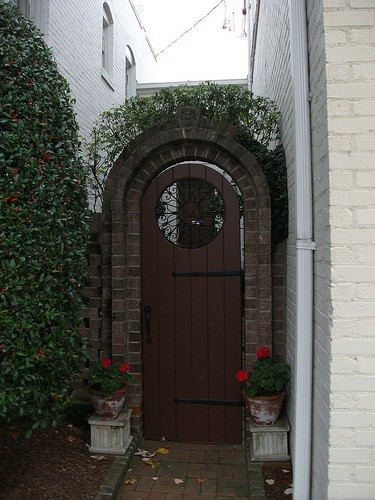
left=86, top=356, right=132, bottom=401
left=235, top=346, right=291, bottom=398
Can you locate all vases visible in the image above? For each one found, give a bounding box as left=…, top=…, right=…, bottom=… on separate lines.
left=88, top=383, right=129, bottom=421
left=246, top=391, right=285, bottom=426
